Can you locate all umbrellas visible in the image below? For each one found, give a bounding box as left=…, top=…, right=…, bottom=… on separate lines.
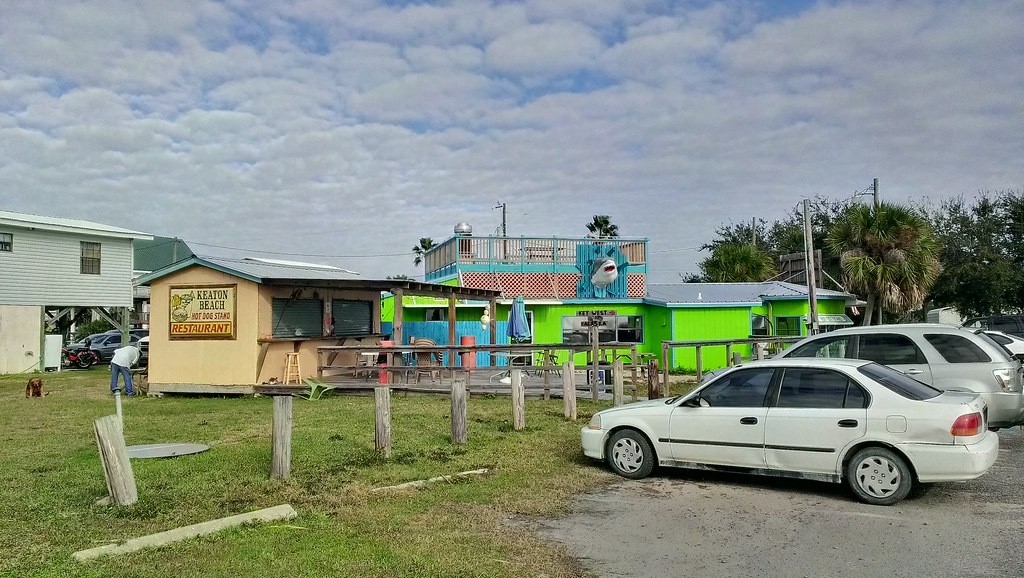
left=505, top=297, right=530, bottom=343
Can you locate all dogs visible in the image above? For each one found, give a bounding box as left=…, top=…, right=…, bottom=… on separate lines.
left=26, top=377, right=50, bottom=400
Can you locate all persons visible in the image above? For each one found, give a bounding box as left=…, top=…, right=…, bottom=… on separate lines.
left=110, top=346, right=143, bottom=396
left=45, top=322, right=52, bottom=332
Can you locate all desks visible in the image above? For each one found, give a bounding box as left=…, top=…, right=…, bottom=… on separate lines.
left=115, top=368, right=145, bottom=396
left=602, top=354, right=656, bottom=366
left=488, top=355, right=532, bottom=385
left=360, top=352, right=411, bottom=382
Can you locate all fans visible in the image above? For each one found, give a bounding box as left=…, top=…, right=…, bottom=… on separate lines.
left=285, top=288, right=303, bottom=307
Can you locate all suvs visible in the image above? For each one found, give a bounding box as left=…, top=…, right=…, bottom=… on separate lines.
left=960, top=315, right=1024, bottom=337
left=702, top=322, right=1024, bottom=435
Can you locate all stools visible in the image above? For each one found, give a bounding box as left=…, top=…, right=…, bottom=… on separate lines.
left=283, top=353, right=301, bottom=385
left=353, top=354, right=372, bottom=379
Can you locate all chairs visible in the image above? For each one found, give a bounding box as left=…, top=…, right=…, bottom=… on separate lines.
left=136, top=365, right=148, bottom=397
left=411, top=338, right=444, bottom=383
left=534, top=344, right=563, bottom=379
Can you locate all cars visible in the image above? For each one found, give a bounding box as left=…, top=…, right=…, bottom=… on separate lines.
left=103, top=329, right=149, bottom=338
left=577, top=356, right=1000, bottom=506
left=886, top=330, right=1024, bottom=368
left=137, top=335, right=150, bottom=361
left=65, top=333, right=142, bottom=361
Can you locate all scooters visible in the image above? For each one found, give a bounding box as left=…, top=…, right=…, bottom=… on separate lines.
left=60, top=338, right=99, bottom=369
